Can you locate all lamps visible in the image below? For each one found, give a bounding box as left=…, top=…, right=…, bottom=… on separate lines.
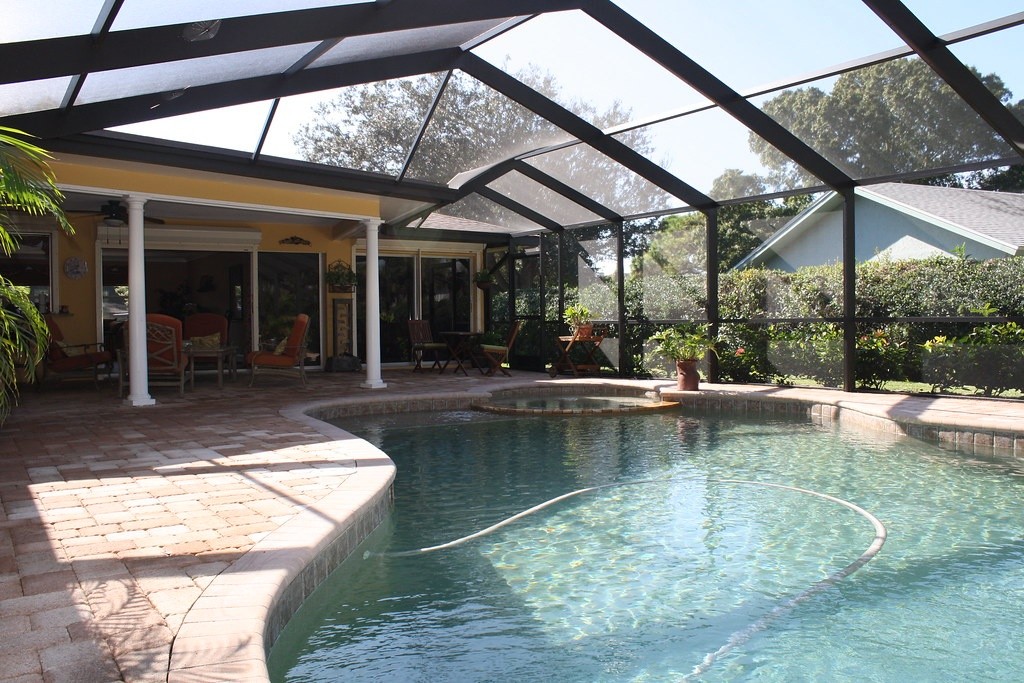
left=104, top=217, right=124, bottom=245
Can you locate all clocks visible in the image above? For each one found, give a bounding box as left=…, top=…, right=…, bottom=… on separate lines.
left=64, top=256, right=89, bottom=279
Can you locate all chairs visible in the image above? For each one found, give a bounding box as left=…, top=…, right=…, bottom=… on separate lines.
left=2, top=330, right=39, bottom=384
left=245, top=314, right=311, bottom=388
left=116, top=314, right=195, bottom=398
left=408, top=319, right=446, bottom=374
left=184, top=314, right=231, bottom=380
left=40, top=315, right=114, bottom=392
left=474, top=319, right=522, bottom=377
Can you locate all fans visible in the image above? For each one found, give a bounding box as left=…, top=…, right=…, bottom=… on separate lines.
left=66, top=200, right=165, bottom=226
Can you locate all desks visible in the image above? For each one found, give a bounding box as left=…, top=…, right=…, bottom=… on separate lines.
left=548, top=327, right=609, bottom=377
left=188, top=344, right=238, bottom=391
left=438, top=331, right=484, bottom=377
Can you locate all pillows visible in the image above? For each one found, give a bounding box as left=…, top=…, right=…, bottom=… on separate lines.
left=273, top=337, right=288, bottom=356
left=56, top=341, right=84, bottom=357
left=190, top=332, right=221, bottom=349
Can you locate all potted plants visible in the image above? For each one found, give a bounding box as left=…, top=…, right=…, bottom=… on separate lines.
left=562, top=304, right=593, bottom=338
left=472, top=269, right=498, bottom=289
left=647, top=320, right=723, bottom=391
left=323, top=266, right=359, bottom=292
